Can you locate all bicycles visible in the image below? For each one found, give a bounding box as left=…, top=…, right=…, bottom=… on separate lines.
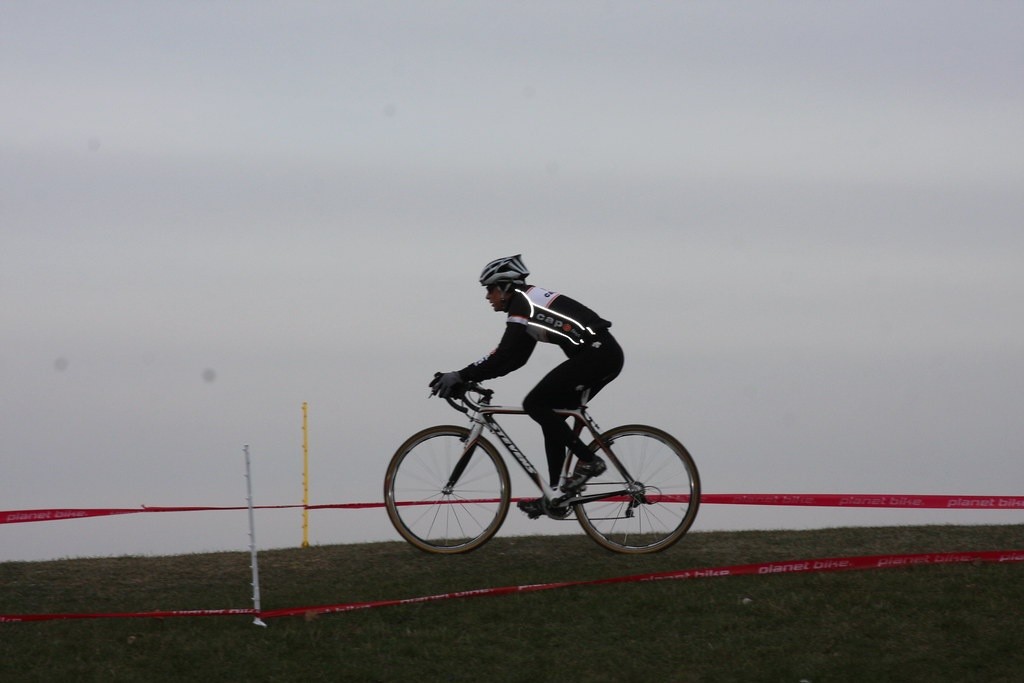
left=382, top=383, right=702, bottom=555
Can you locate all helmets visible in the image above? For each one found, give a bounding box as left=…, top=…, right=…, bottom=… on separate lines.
left=479, top=253, right=531, bottom=285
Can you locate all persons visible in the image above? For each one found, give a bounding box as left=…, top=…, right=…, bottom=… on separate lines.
left=429, top=255, right=625, bottom=517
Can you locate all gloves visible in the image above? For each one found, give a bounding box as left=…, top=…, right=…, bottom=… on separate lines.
left=428, top=371, right=468, bottom=400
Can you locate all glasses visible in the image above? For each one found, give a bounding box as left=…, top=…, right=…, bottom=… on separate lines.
left=485, top=284, right=498, bottom=295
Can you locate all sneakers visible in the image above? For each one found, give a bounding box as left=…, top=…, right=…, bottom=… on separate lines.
left=560, top=458, right=607, bottom=494
left=518, top=499, right=567, bottom=518
